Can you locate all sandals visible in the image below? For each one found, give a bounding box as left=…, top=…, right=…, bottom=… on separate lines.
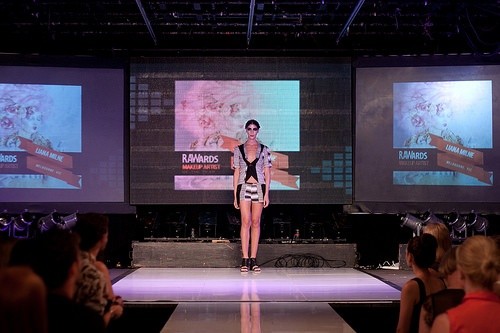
left=240, top=258, right=261, bottom=272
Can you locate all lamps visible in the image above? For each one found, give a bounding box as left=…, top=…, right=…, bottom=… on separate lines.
left=1, top=210, right=82, bottom=236
left=400, top=209, right=488, bottom=234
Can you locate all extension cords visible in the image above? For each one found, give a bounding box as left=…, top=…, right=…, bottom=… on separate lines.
left=382, top=266, right=400, bottom=270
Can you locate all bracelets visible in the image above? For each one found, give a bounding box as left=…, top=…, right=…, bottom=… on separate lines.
left=109, top=309, right=114, bottom=317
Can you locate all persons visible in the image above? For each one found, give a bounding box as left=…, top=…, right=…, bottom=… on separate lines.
left=418, top=246, right=465, bottom=333
left=423, top=222, right=451, bottom=277
left=396, top=233, right=447, bottom=333
left=71, top=213, right=124, bottom=328
left=240, top=272, right=260, bottom=333
left=233, top=120, right=272, bottom=271
left=0, top=230, right=46, bottom=333
left=26, top=229, right=107, bottom=333
left=429, top=235, right=500, bottom=333
left=95, top=261, right=123, bottom=314
